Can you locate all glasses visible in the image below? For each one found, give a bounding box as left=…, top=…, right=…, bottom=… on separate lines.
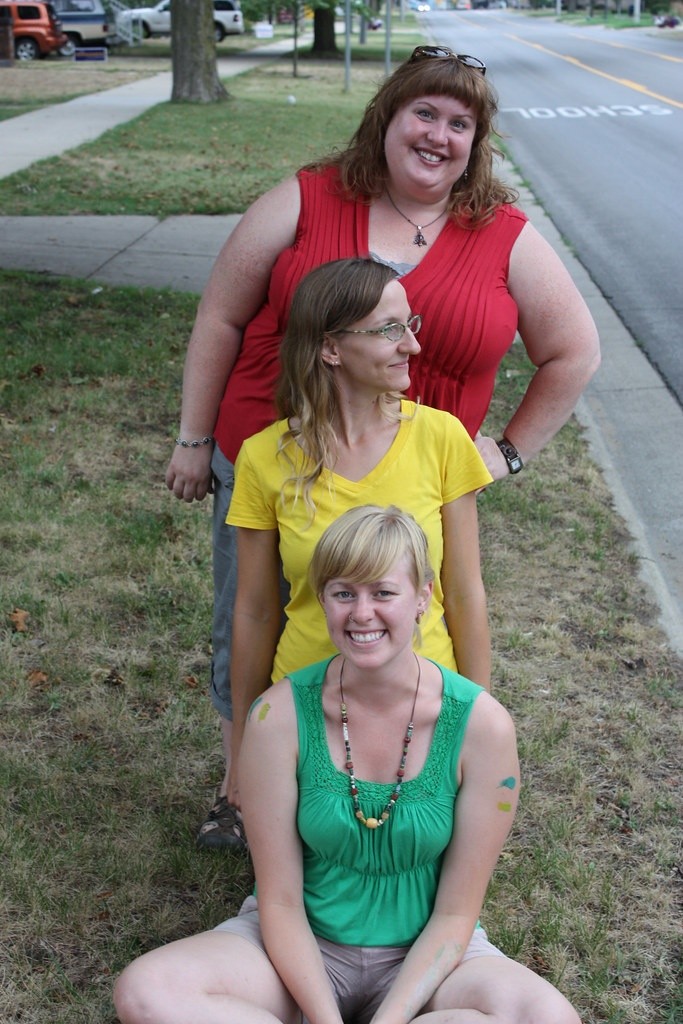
left=333, top=314, right=423, bottom=342
left=405, top=45, right=487, bottom=77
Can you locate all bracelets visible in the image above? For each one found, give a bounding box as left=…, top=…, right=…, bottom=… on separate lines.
left=174, top=436, right=212, bottom=448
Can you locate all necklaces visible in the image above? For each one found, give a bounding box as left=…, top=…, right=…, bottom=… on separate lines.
left=339, top=651, right=421, bottom=828
left=383, top=177, right=452, bottom=247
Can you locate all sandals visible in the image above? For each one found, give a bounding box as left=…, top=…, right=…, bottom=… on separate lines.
left=196, top=794, right=246, bottom=856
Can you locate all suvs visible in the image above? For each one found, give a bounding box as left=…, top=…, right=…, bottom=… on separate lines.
left=0, top=0, right=110, bottom=63
left=116, top=0, right=245, bottom=43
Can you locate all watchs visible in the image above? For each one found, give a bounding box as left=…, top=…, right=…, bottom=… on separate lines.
left=498, top=438, right=523, bottom=474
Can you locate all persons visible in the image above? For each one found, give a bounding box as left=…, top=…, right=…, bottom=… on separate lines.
left=112, top=505, right=584, bottom=1024
left=165, top=44, right=602, bottom=855
left=225, top=259, right=496, bottom=810
